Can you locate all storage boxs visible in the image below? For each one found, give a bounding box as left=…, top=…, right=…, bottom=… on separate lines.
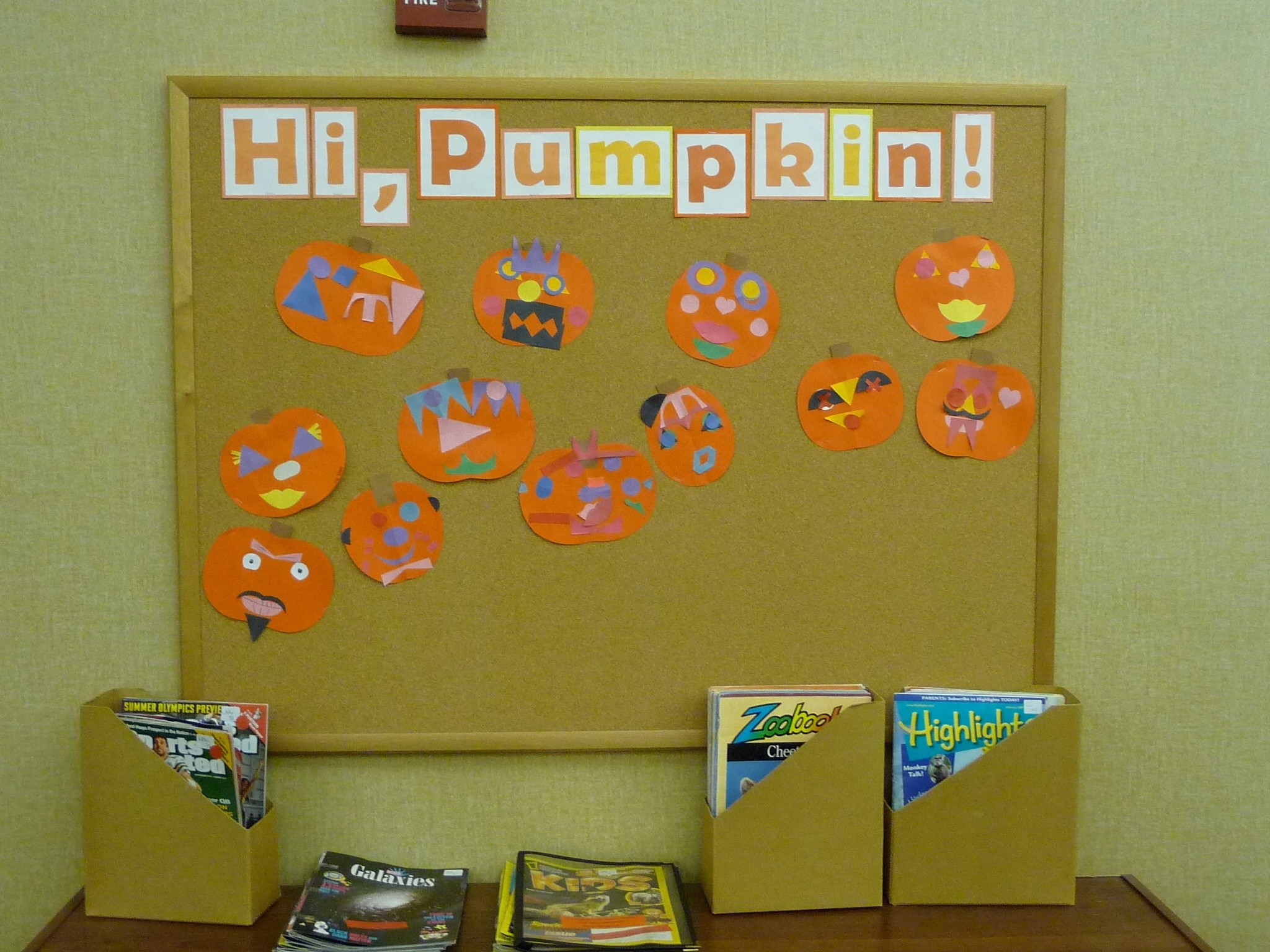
left=884, top=685, right=1083, bottom=907
left=698, top=686, right=885, bottom=916
left=80, top=687, right=282, bottom=925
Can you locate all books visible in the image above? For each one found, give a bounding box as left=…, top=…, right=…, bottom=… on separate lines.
left=892, top=687, right=1066, bottom=812
left=492, top=851, right=701, bottom=952
left=705, top=684, right=871, bottom=818
left=114, top=700, right=269, bottom=827
left=271, top=850, right=469, bottom=952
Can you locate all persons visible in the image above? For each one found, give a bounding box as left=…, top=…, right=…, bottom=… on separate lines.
left=242, top=760, right=264, bottom=803
left=152, top=736, right=201, bottom=792
